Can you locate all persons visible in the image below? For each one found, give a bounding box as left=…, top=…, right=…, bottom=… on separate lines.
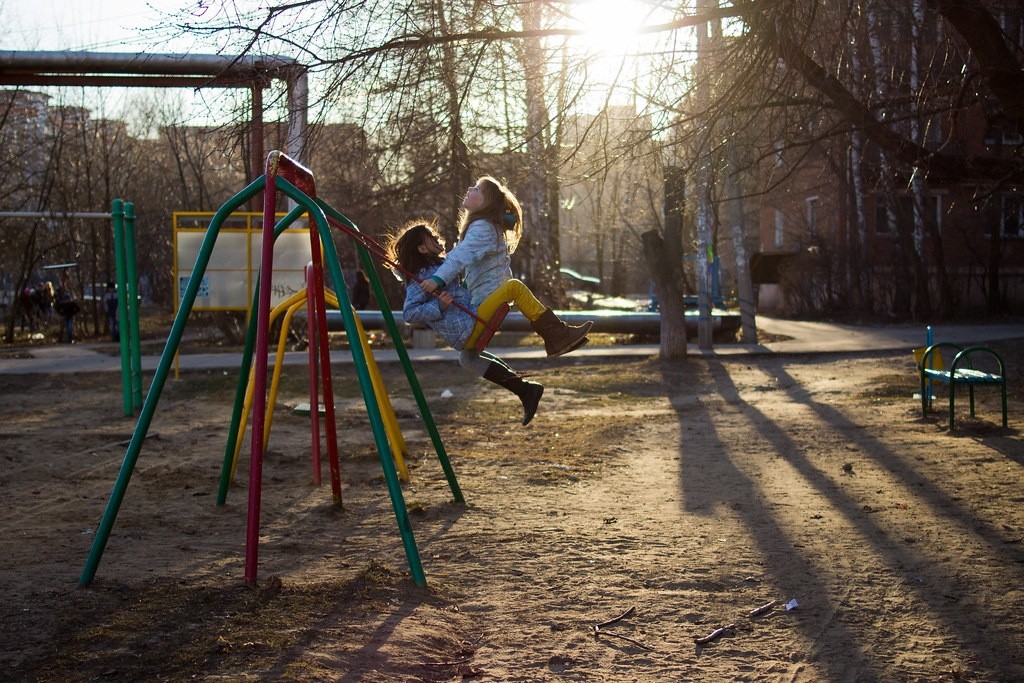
left=54, top=286, right=80, bottom=343
left=20, top=281, right=53, bottom=332
left=352, top=270, right=370, bottom=310
left=105, top=282, right=119, bottom=342
left=383, top=177, right=593, bottom=426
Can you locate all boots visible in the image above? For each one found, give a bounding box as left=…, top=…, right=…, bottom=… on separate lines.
left=530, top=307, right=593, bottom=358
left=483, top=357, right=543, bottom=426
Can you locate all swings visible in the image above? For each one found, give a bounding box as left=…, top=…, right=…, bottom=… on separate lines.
left=324, top=211, right=510, bottom=353
